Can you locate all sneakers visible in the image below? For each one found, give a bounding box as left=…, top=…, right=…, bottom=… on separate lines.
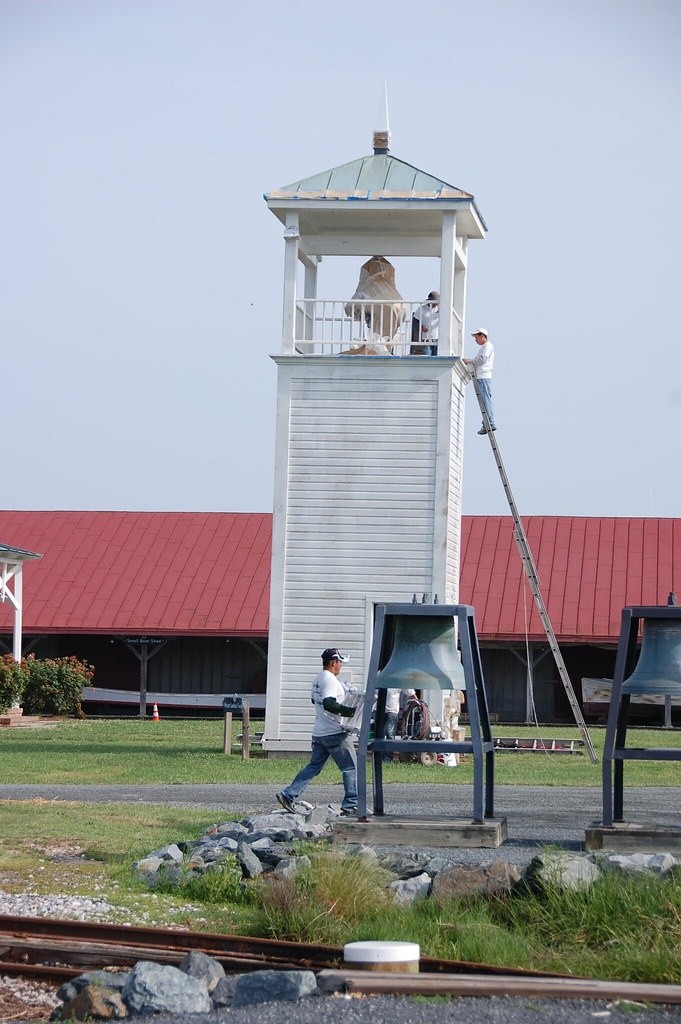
left=340, top=807, right=374, bottom=817
left=276, top=793, right=296, bottom=814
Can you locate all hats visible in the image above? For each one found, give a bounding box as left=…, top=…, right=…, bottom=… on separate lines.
left=321, top=648, right=349, bottom=663
left=428, top=291, right=439, bottom=300
left=471, top=328, right=488, bottom=338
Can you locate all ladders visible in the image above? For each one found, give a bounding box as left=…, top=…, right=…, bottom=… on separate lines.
left=463, top=735, right=586, bottom=757
left=471, top=363, right=601, bottom=768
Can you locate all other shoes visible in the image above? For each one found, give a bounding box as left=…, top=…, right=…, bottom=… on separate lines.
left=383, top=757, right=391, bottom=761
left=477, top=424, right=497, bottom=434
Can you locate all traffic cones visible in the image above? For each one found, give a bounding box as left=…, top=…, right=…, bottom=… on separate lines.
left=152, top=700, right=160, bottom=721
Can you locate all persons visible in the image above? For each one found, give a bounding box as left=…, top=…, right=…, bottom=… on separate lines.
left=412, top=292, right=440, bottom=355
left=444, top=689, right=465, bottom=738
left=371, top=669, right=419, bottom=764
left=276, top=648, right=357, bottom=814
left=462, top=328, right=496, bottom=435
